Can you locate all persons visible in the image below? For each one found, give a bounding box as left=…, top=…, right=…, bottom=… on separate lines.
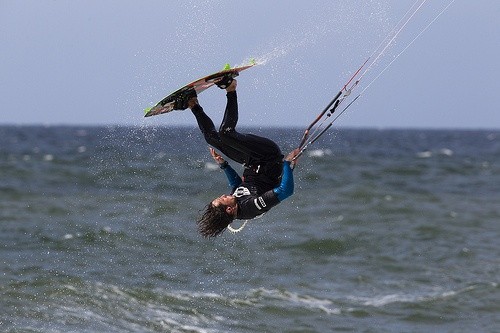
left=172, top=72, right=303, bottom=236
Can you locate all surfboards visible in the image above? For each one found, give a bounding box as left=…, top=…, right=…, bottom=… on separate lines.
left=143, top=57, right=258, bottom=119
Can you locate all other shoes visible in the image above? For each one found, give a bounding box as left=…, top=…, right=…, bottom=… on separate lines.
left=173, top=91, right=196, bottom=110
left=214, top=76, right=234, bottom=89
